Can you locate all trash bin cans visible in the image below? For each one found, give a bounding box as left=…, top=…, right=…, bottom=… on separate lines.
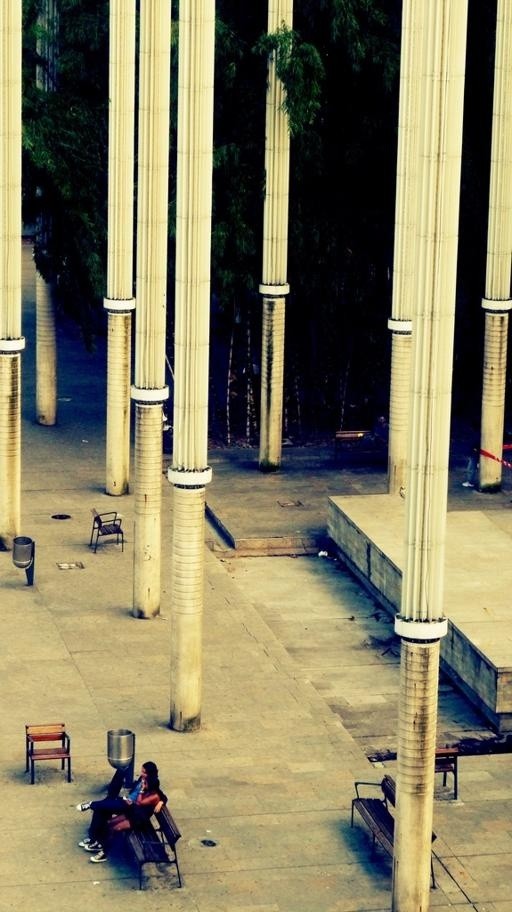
left=13, top=537, right=35, bottom=586
left=107, top=730, right=135, bottom=789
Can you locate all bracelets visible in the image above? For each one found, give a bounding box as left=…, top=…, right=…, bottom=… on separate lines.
left=138, top=790, right=144, bottom=794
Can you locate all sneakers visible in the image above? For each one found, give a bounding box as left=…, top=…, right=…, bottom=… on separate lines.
left=84, top=840, right=104, bottom=851
left=90, top=851, right=107, bottom=863
left=78, top=838, right=91, bottom=847
left=76, top=800, right=92, bottom=812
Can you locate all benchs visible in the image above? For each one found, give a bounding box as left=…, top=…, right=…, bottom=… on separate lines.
left=122, top=805, right=181, bottom=890
left=352, top=776, right=438, bottom=890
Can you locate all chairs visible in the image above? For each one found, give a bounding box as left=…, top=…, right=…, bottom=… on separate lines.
left=24, top=722, right=72, bottom=783
left=435, top=747, right=459, bottom=800
left=89, top=508, right=124, bottom=553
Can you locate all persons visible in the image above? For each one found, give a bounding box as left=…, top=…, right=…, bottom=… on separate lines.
left=84, top=774, right=160, bottom=863
left=77, top=762, right=168, bottom=847
